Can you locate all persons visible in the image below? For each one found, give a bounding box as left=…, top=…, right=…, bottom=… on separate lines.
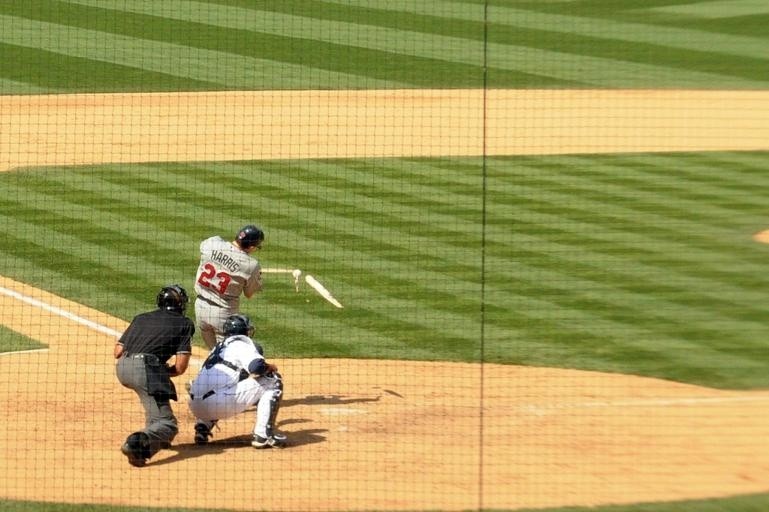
left=193, top=224, right=264, bottom=350
left=184, top=314, right=288, bottom=449
left=113, top=284, right=196, bottom=467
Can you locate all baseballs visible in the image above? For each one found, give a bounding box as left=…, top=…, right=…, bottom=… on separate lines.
left=293, top=270, right=301, bottom=277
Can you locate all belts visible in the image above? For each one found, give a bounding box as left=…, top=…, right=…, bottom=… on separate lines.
left=189, top=391, right=214, bottom=400
left=123, top=352, right=144, bottom=359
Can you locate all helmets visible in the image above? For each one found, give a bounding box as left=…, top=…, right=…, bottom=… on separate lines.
left=236, top=225, right=264, bottom=251
left=158, top=285, right=188, bottom=314
left=222, top=314, right=255, bottom=337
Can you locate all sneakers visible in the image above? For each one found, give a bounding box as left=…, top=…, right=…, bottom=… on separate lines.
left=122, top=442, right=146, bottom=466
left=194, top=424, right=213, bottom=443
left=251, top=436, right=287, bottom=448
